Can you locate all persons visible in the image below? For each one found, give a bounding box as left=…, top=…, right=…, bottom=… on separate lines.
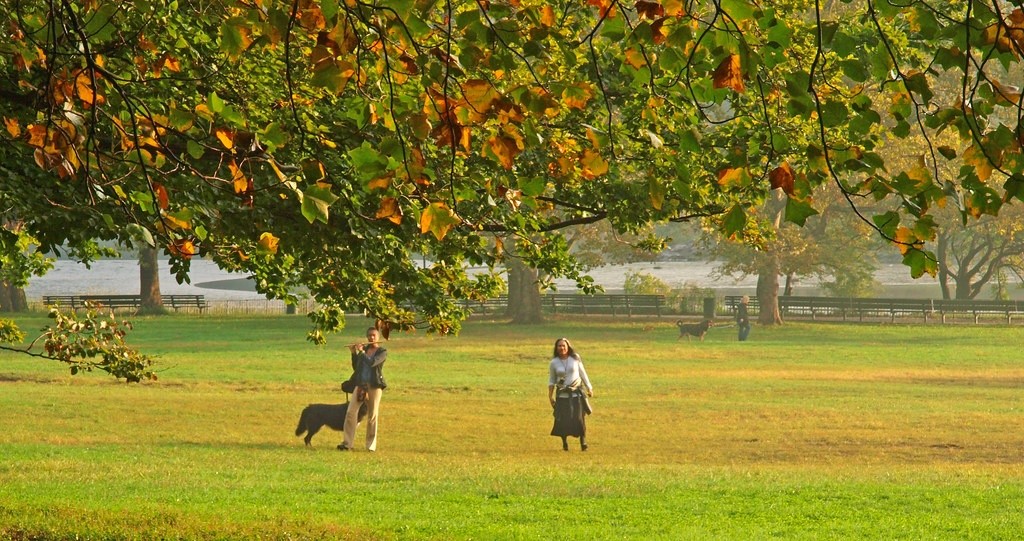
left=336, top=328, right=387, bottom=452
left=736, top=295, right=750, bottom=340
left=549, top=337, right=594, bottom=448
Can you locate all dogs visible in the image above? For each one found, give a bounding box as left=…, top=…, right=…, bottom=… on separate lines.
left=295, top=398, right=368, bottom=445
left=676, top=320, right=715, bottom=341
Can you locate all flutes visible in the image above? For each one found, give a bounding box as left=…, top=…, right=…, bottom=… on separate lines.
left=344, top=341, right=384, bottom=347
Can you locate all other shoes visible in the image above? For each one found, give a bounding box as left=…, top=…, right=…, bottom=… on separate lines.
left=563, top=443, right=589, bottom=451
left=337, top=444, right=348, bottom=450
